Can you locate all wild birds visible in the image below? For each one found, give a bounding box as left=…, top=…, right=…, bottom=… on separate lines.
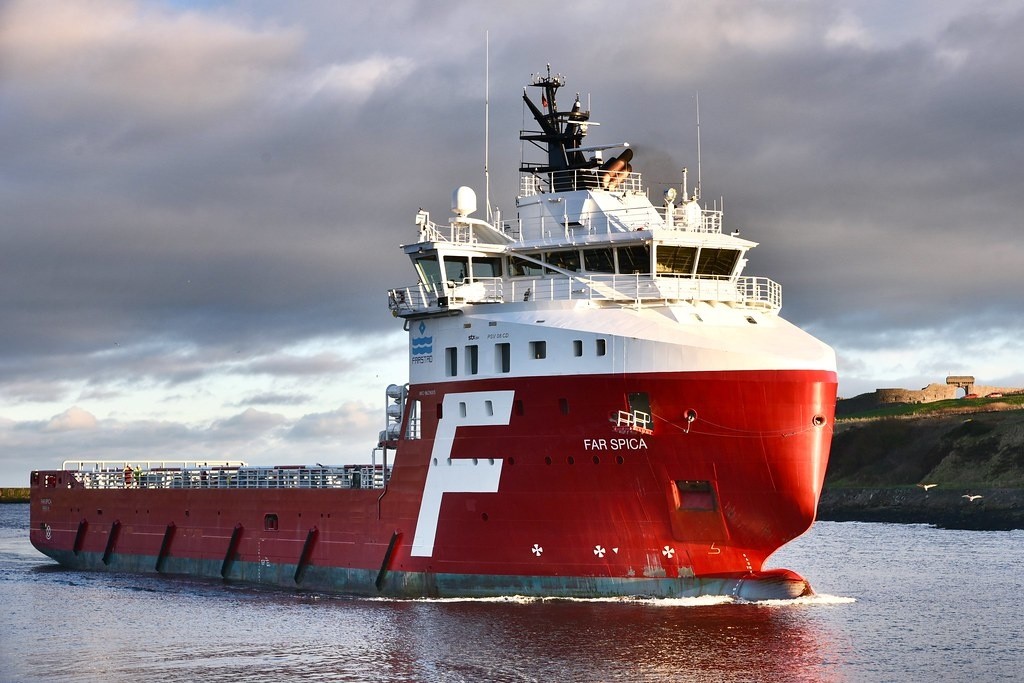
left=916, top=482, right=939, bottom=493
left=961, top=494, right=984, bottom=502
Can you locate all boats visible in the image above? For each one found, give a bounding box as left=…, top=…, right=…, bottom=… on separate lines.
left=29, top=29, right=839, bottom=600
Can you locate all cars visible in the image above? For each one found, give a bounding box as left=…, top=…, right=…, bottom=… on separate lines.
left=986, top=392, right=1004, bottom=398
left=962, top=394, right=979, bottom=399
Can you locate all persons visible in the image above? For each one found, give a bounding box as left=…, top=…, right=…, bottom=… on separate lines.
left=217, top=465, right=225, bottom=488
left=133, top=464, right=144, bottom=486
left=199, top=463, right=212, bottom=489
left=123, top=464, right=133, bottom=488
left=352, top=464, right=361, bottom=489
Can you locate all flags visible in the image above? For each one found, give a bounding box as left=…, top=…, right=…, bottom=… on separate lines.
left=541, top=94, right=548, bottom=107
